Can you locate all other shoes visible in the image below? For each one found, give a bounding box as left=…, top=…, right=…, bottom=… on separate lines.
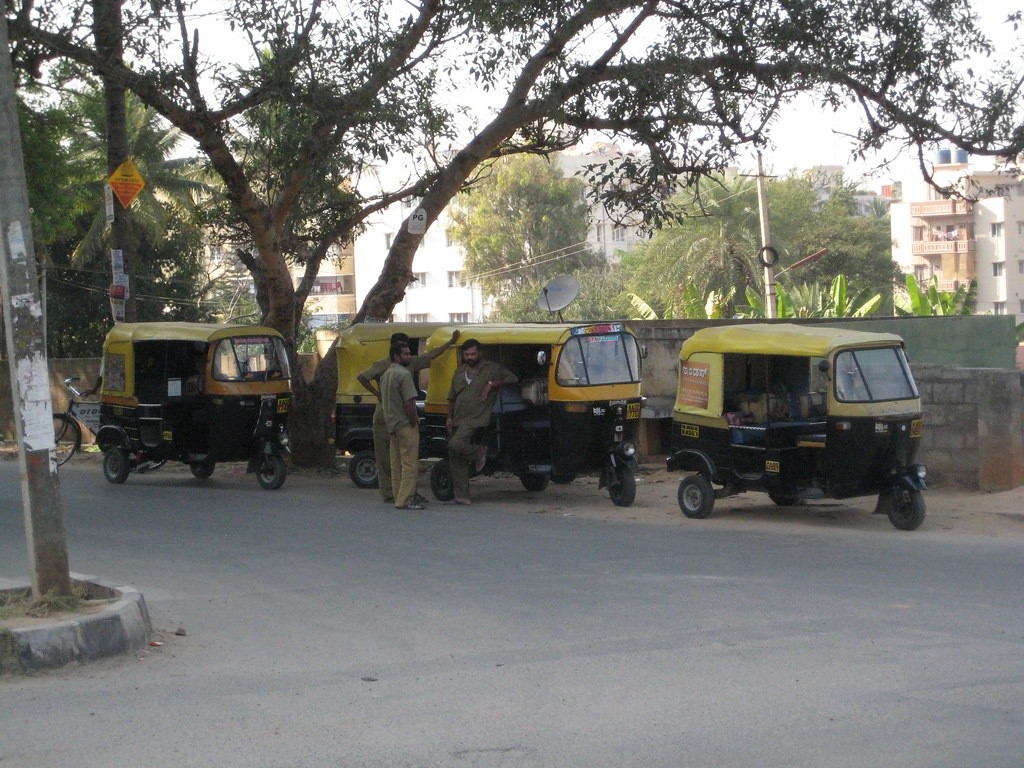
left=398, top=501, right=424, bottom=510
left=456, top=497, right=471, bottom=505
left=383, top=497, right=395, bottom=503
left=413, top=493, right=429, bottom=503
left=475, top=446, right=487, bottom=472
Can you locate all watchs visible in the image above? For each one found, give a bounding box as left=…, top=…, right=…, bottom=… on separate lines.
left=488, top=379, right=494, bottom=387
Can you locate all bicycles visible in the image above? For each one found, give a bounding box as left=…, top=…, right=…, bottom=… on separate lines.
left=52, top=376, right=100, bottom=467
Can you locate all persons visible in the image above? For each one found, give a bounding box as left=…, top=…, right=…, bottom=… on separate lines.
left=379, top=341, right=423, bottom=510
left=445, top=340, right=519, bottom=506
left=81, top=355, right=104, bottom=399
left=357, top=332, right=461, bottom=504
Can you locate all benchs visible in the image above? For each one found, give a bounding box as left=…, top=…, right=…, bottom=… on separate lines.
left=134, top=367, right=204, bottom=446
left=484, top=387, right=547, bottom=461
left=723, top=390, right=829, bottom=487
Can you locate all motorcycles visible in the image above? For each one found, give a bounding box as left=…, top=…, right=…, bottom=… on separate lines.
left=334, top=318, right=649, bottom=508
left=92, top=321, right=295, bottom=490
left=665, top=322, right=930, bottom=531
